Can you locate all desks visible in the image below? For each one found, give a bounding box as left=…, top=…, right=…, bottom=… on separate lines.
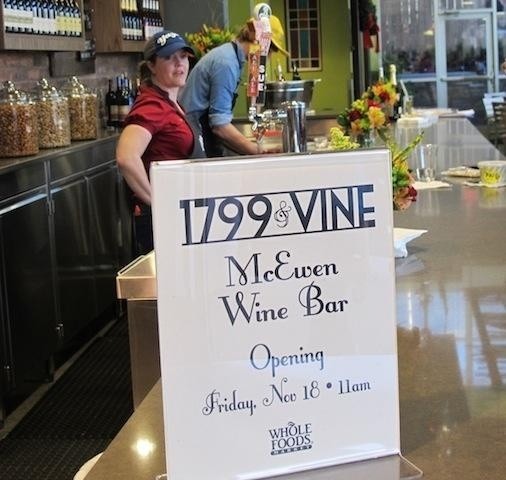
left=80, top=107, right=506, bottom=479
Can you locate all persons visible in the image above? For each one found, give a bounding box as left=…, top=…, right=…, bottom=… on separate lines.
left=177, top=14, right=289, bottom=158
left=116, top=31, right=195, bottom=254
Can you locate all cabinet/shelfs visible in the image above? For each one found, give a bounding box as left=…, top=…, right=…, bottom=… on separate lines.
left=0, top=128, right=133, bottom=424
left=0, top=0, right=86, bottom=51
left=88, top=0, right=164, bottom=54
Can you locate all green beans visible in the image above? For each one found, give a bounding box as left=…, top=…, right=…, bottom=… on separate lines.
left=0, top=94, right=97, bottom=157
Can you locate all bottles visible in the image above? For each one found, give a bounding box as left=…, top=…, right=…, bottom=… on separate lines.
left=3, top=0, right=83, bottom=37
left=378, top=63, right=401, bottom=123
left=105, top=72, right=141, bottom=129
left=0, top=75, right=100, bottom=159
left=120, top=0, right=165, bottom=41
left=276, top=58, right=301, bottom=81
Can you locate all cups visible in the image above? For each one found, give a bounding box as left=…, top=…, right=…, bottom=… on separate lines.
left=477, top=160, right=506, bottom=183
left=414, top=144, right=439, bottom=182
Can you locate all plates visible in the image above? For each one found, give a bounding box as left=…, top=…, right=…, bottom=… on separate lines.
left=463, top=182, right=506, bottom=188
left=412, top=181, right=451, bottom=191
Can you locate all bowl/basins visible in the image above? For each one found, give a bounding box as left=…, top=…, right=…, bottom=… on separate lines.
left=266, top=79, right=315, bottom=108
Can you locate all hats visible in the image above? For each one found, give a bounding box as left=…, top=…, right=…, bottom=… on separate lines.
left=143, top=31, right=196, bottom=60
left=250, top=15, right=291, bottom=59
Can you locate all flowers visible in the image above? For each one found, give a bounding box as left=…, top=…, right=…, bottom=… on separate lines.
left=376, top=127, right=425, bottom=201
left=336, top=78, right=398, bottom=137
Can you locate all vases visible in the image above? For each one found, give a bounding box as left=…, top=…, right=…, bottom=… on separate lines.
left=361, top=127, right=376, bottom=149
left=393, top=197, right=412, bottom=211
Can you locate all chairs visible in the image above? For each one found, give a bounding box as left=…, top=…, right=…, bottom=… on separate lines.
left=493, top=103, right=506, bottom=148
